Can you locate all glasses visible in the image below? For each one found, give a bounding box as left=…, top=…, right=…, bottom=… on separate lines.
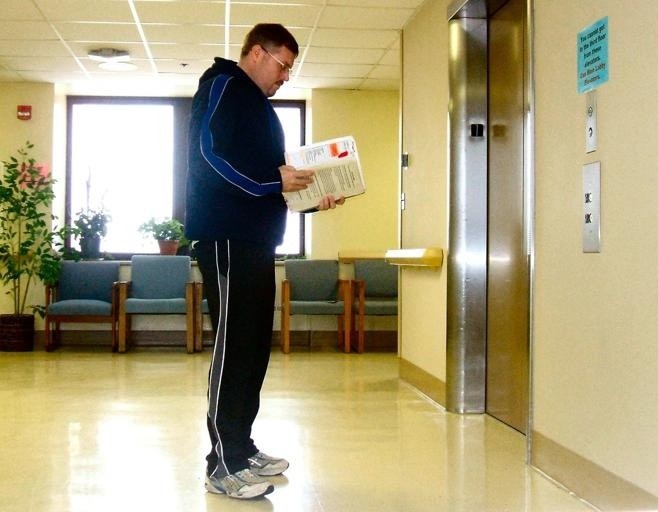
left=255, top=42, right=293, bottom=74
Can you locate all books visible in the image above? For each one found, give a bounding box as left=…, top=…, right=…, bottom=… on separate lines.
left=279, top=133, right=367, bottom=214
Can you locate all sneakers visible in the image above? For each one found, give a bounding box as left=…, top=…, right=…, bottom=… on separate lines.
left=204, top=451, right=290, bottom=500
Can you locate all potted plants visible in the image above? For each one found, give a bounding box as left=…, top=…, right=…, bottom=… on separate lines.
left=70, top=207, right=110, bottom=258
left=0, top=139, right=69, bottom=353
left=138, top=216, right=192, bottom=255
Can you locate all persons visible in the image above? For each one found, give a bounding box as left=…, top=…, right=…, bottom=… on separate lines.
left=182, top=21, right=347, bottom=502
